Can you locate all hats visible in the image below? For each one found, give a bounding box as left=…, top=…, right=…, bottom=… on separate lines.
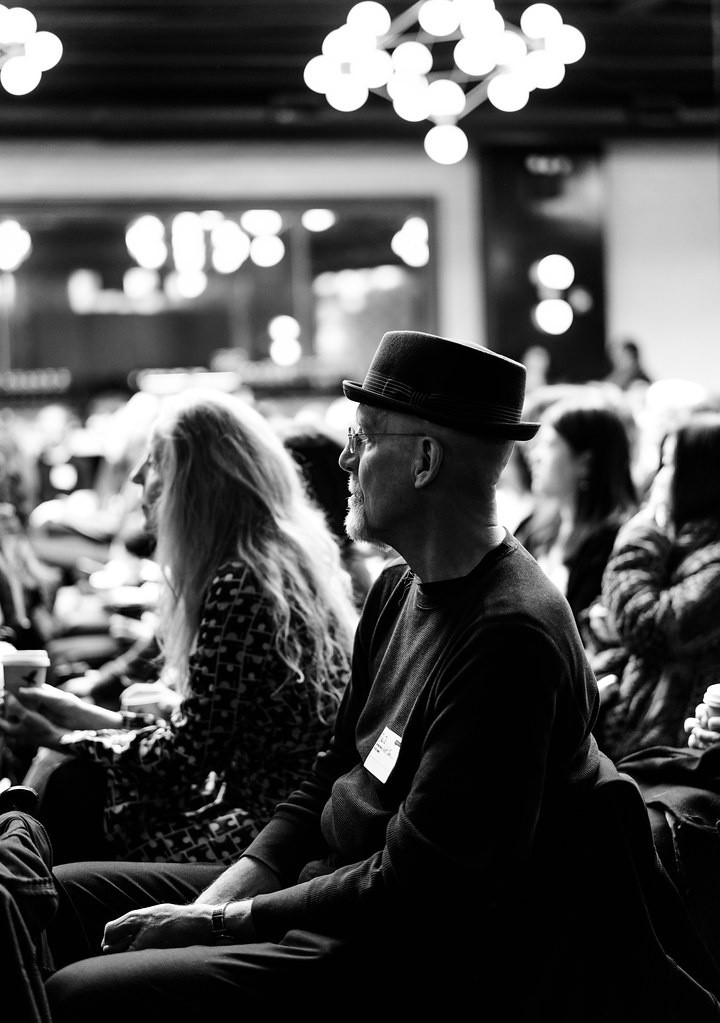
left=343, top=331, right=538, bottom=440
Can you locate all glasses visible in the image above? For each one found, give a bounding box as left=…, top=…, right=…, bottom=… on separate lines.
left=348, top=425, right=444, bottom=453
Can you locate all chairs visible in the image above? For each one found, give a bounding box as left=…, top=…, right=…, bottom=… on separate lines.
left=583, top=751, right=664, bottom=1021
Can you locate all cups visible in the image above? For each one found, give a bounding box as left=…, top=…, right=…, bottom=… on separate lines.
left=2, top=647, right=52, bottom=712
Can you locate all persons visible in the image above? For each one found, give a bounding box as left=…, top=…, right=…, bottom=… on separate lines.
left=0, top=330, right=720, bottom=1023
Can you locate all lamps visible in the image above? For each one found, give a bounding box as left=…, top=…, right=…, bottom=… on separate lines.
left=0, top=4, right=60, bottom=95
left=305, top=2, right=586, bottom=164
